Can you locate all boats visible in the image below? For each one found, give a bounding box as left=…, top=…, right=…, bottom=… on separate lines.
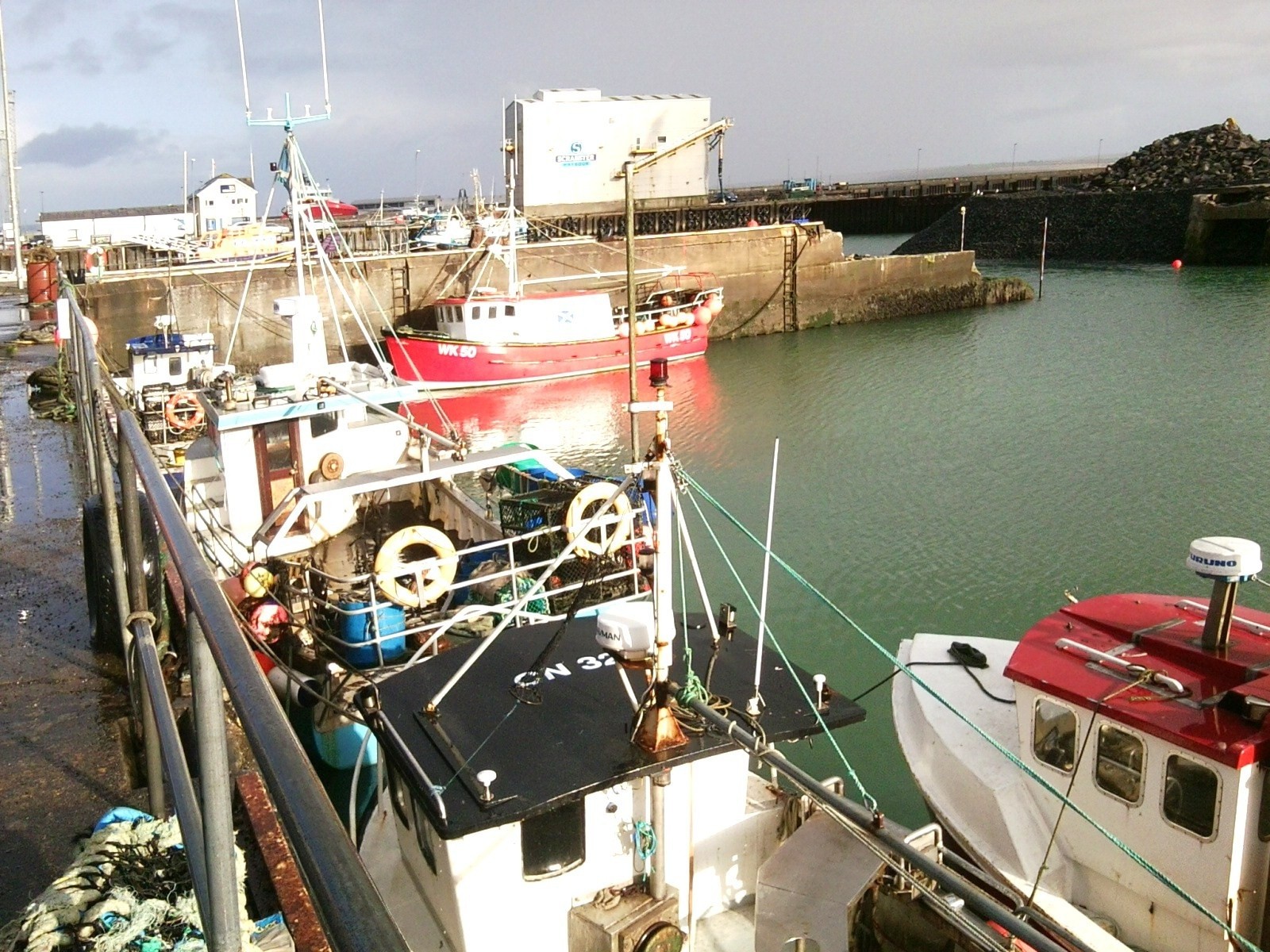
left=374, top=92, right=724, bottom=392
left=880, top=528, right=1270, bottom=952
left=109, top=2, right=1152, bottom=952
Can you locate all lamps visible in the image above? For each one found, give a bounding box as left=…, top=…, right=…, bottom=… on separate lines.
left=812, top=674, right=826, bottom=709
left=476, top=770, right=497, bottom=801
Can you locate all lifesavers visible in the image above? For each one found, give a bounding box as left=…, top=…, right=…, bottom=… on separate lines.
left=567, top=483, right=633, bottom=559
left=82, top=490, right=162, bottom=646
left=166, top=393, right=204, bottom=429
left=373, top=526, right=458, bottom=606
left=471, top=287, right=498, bottom=293
